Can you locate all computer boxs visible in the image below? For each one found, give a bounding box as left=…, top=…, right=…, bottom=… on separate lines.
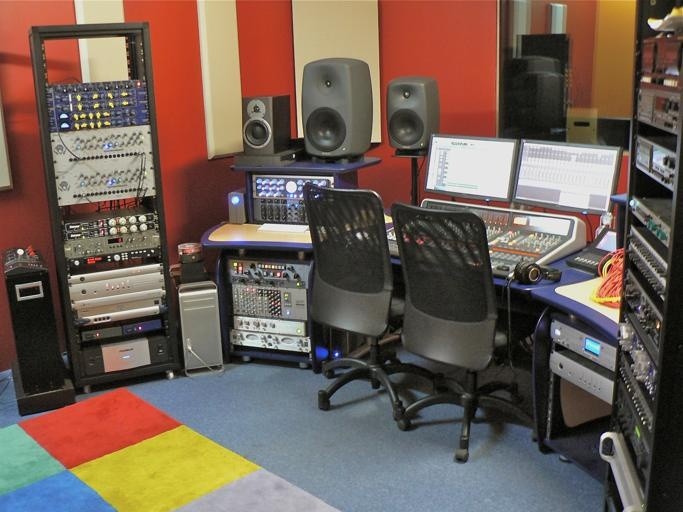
left=173, top=260, right=225, bottom=377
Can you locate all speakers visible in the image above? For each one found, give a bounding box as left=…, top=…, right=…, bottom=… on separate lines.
left=387, top=78, right=439, bottom=155
left=510, top=55, right=562, bottom=73
left=241, top=96, right=290, bottom=155
left=301, top=58, right=373, bottom=167
left=228, top=186, right=248, bottom=225
left=507, top=76, right=567, bottom=141
left=521, top=33, right=570, bottom=63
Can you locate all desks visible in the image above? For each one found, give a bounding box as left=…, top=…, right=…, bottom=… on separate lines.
left=200, top=155, right=629, bottom=486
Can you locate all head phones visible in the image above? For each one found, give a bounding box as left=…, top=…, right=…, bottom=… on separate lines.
left=513, top=259, right=563, bottom=286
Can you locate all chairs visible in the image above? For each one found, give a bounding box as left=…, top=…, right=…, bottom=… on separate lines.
left=391, top=202, right=535, bottom=462
left=302, top=182, right=444, bottom=431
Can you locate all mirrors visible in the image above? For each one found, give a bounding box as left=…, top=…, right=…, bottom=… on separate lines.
left=496, top=0, right=568, bottom=140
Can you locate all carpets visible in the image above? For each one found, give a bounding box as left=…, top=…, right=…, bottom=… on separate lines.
left=0, top=388, right=345, bottom=512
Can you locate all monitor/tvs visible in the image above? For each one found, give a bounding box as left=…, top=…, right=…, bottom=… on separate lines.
left=512, top=138, right=622, bottom=220
left=424, top=132, right=519, bottom=212
left=595, top=117, right=632, bottom=149
